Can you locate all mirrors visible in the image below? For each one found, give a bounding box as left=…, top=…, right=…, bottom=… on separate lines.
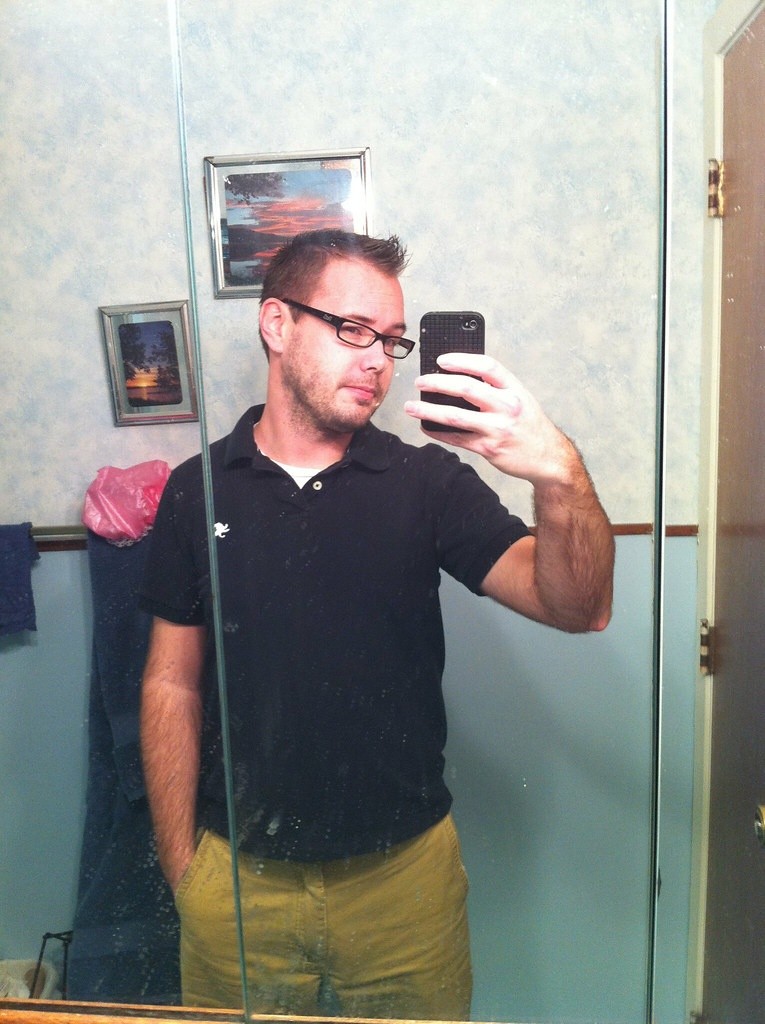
left=0, top=0, right=720, bottom=1024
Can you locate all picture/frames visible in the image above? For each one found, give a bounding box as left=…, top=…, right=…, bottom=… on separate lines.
left=98, top=297, right=201, bottom=427
left=204, top=147, right=373, bottom=300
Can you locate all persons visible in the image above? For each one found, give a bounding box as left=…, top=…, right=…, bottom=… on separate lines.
left=141, top=228, right=616, bottom=1022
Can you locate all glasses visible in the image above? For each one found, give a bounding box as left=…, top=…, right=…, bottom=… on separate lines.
left=282, top=297, right=415, bottom=359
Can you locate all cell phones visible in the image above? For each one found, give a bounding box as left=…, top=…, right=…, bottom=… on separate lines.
left=420, top=311, right=485, bottom=436
